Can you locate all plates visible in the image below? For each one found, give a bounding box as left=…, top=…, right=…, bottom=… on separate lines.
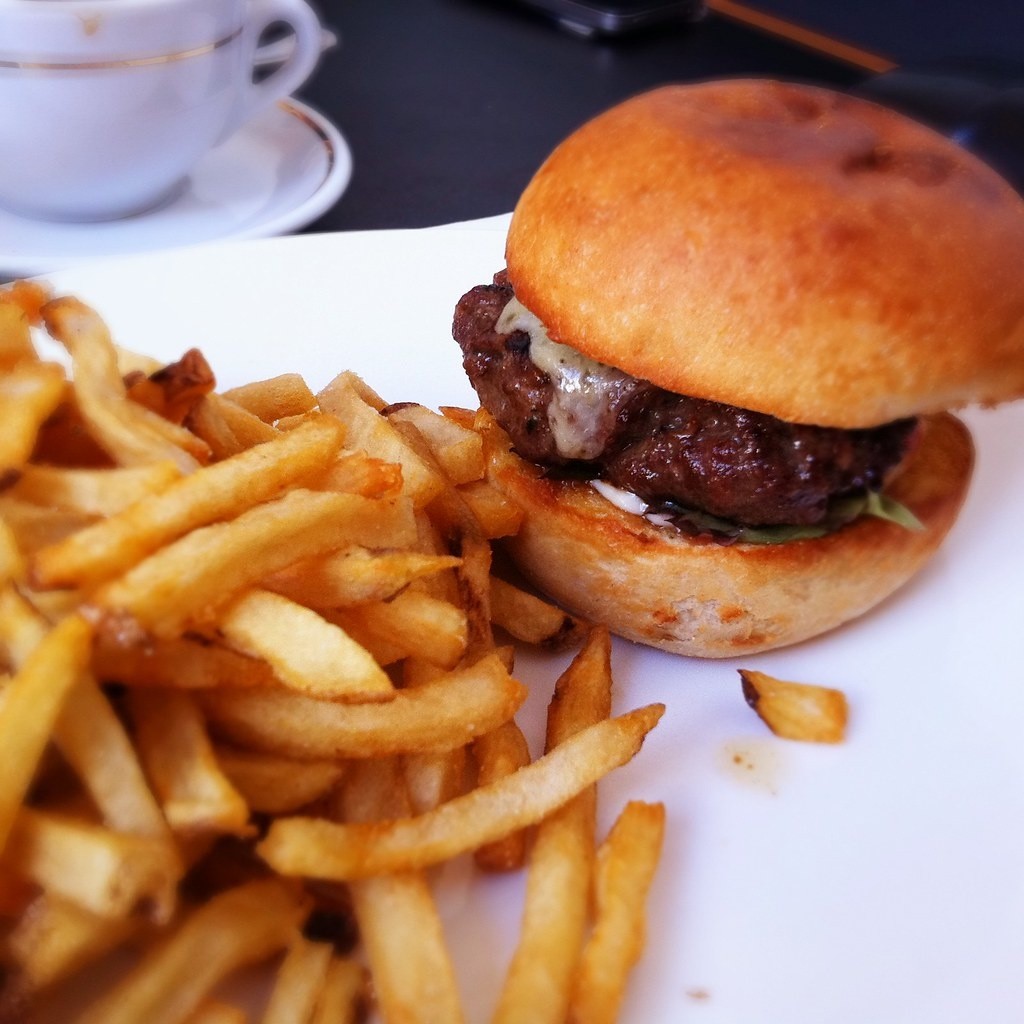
left=0, top=207, right=1024, bottom=1024
left=0, top=97, right=353, bottom=280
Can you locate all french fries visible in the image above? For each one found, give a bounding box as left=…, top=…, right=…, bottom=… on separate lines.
left=1, top=279, right=847, bottom=1024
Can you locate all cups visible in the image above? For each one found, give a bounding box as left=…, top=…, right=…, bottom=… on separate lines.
left=0, top=0, right=324, bottom=224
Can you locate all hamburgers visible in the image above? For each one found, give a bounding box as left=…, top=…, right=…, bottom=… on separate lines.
left=441, top=76, right=1024, bottom=658
